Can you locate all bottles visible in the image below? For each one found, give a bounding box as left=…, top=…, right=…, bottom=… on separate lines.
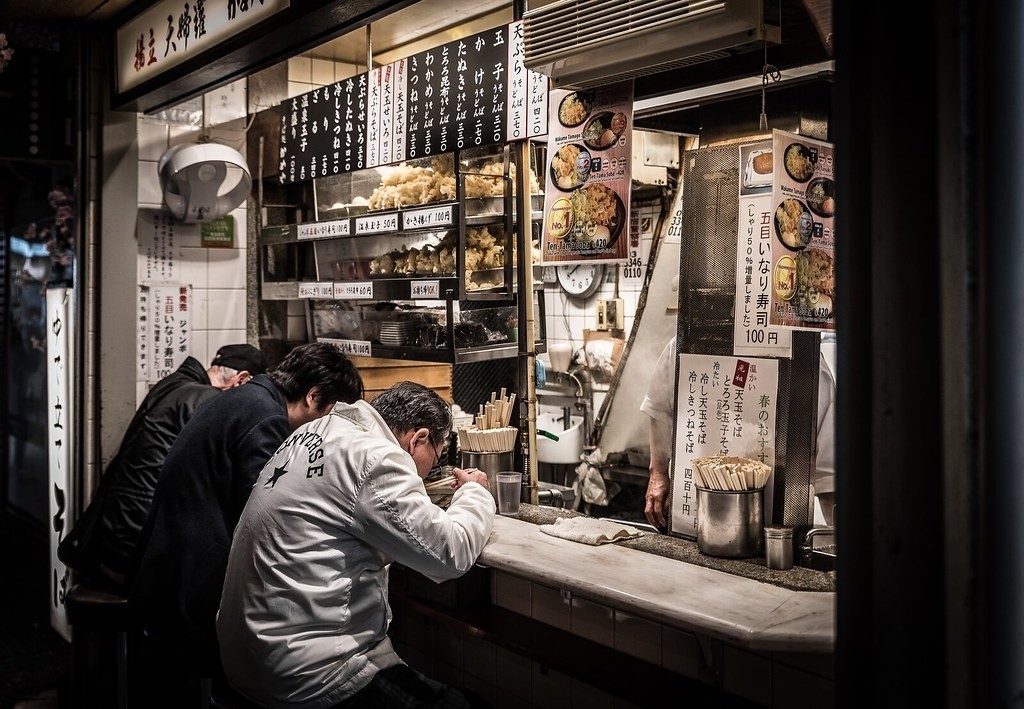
left=764, top=525, right=794, bottom=570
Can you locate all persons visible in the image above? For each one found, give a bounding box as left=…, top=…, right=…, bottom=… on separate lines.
left=130, top=345, right=360, bottom=709
left=639, top=336, right=836, bottom=529
left=58, top=340, right=295, bottom=597
left=215, top=382, right=498, bottom=709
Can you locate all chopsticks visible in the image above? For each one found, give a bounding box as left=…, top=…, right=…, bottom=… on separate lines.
left=458, top=388, right=519, bottom=452
left=690, top=455, right=772, bottom=491
left=425, top=468, right=478, bottom=490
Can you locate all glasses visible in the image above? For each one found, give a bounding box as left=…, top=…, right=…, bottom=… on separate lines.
left=414, top=428, right=443, bottom=479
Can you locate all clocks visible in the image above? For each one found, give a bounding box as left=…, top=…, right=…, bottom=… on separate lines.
left=556, top=264, right=604, bottom=299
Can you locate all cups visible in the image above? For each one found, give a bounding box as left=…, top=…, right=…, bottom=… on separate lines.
left=460, top=448, right=515, bottom=506
left=496, top=471, right=522, bottom=515
left=694, top=483, right=767, bottom=559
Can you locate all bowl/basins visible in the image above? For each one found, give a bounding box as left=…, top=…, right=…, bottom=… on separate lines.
left=583, top=111, right=622, bottom=151
left=548, top=197, right=574, bottom=239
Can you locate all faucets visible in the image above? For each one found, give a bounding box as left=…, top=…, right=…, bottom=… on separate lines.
left=556, top=406, right=572, bottom=431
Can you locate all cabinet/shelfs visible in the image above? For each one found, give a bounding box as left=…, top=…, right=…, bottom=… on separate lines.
left=259, top=141, right=547, bottom=365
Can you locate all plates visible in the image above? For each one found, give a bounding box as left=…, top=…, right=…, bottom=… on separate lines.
left=558, top=92, right=592, bottom=128
left=774, top=198, right=813, bottom=252
left=563, top=187, right=626, bottom=257
left=743, top=149, right=773, bottom=188
left=379, top=320, right=411, bottom=347
left=805, top=177, right=836, bottom=218
left=773, top=255, right=797, bottom=301
left=550, top=143, right=591, bottom=192
left=784, top=143, right=814, bottom=183
left=790, top=251, right=834, bottom=324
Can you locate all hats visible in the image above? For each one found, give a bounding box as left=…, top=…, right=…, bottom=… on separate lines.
left=211, top=344, right=269, bottom=376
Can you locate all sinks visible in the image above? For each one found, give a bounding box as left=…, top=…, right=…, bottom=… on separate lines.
left=535, top=412, right=585, bottom=465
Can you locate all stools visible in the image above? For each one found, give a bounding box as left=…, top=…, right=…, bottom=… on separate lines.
left=66, top=580, right=136, bottom=709
